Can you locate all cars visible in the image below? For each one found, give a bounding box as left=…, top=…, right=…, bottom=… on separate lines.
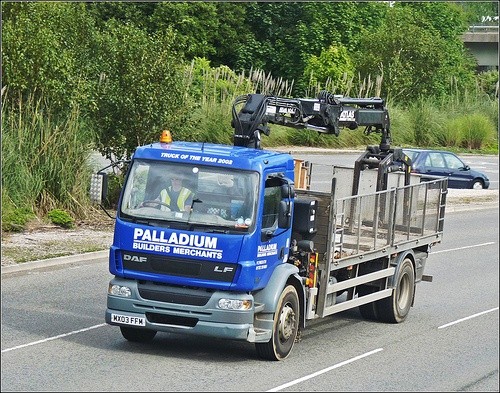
left=392, top=148, right=490, bottom=190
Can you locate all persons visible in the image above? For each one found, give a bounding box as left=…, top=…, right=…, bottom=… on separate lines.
left=139, top=170, right=196, bottom=212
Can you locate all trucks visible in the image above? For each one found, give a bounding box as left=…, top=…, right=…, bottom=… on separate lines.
left=89, top=95, right=446, bottom=360
